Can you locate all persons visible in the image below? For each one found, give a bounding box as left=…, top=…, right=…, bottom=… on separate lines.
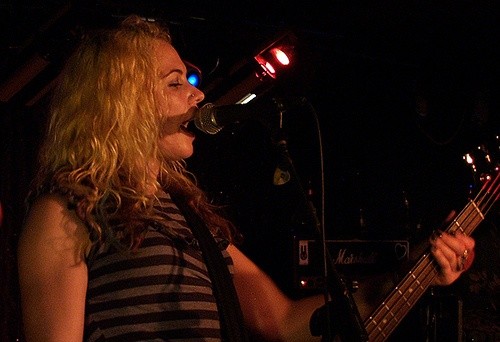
left=15, top=13, right=475, bottom=342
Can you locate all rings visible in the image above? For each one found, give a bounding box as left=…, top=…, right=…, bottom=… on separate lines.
left=458, top=249, right=470, bottom=265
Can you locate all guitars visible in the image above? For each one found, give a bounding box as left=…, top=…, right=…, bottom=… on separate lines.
left=309, top=136, right=500, bottom=342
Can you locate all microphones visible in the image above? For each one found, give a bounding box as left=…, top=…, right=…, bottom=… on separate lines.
left=193, top=96, right=307, bottom=134
